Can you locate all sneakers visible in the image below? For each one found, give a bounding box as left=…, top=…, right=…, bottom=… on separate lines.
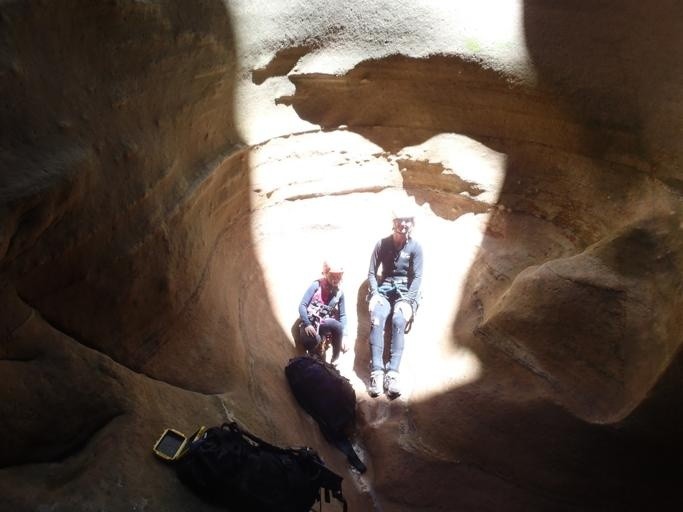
left=368, top=370, right=401, bottom=397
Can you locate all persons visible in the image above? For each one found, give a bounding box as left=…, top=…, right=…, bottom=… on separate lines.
left=364, top=213, right=424, bottom=398
left=298, top=260, right=349, bottom=363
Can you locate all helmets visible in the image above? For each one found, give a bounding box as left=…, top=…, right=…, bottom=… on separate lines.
left=323, top=258, right=344, bottom=273
left=392, top=206, right=415, bottom=219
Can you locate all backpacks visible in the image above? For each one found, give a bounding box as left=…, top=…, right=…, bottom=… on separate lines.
left=284, top=356, right=367, bottom=474
left=178, top=423, right=348, bottom=511
